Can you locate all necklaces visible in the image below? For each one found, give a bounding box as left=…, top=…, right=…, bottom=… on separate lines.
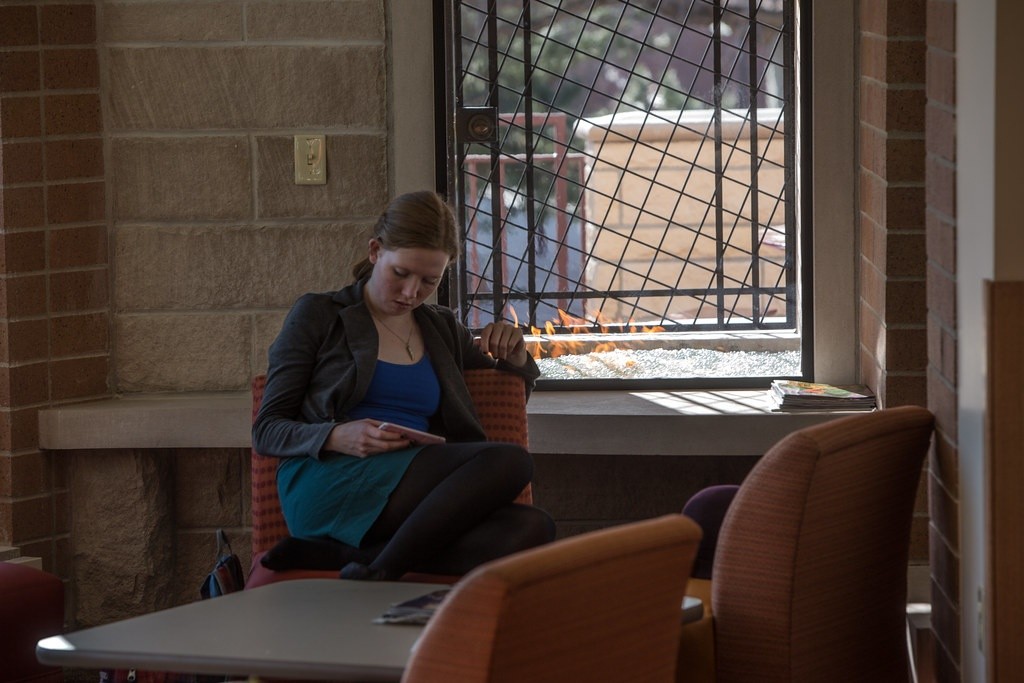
left=374, top=314, right=414, bottom=362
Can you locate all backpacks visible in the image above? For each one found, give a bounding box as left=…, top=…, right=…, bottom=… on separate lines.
left=200, top=529, right=243, bottom=600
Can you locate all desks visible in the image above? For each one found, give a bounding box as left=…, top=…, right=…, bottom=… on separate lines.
left=31, top=576, right=701, bottom=683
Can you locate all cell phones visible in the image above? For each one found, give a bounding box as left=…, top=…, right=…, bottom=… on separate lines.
left=378, top=423, right=446, bottom=445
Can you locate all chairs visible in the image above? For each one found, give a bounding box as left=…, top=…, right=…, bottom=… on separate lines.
left=708, top=405, right=938, bottom=682
left=397, top=510, right=703, bottom=683
left=242, top=363, right=533, bottom=592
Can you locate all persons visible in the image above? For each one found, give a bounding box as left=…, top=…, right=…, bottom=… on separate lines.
left=250, top=190, right=556, bottom=582
